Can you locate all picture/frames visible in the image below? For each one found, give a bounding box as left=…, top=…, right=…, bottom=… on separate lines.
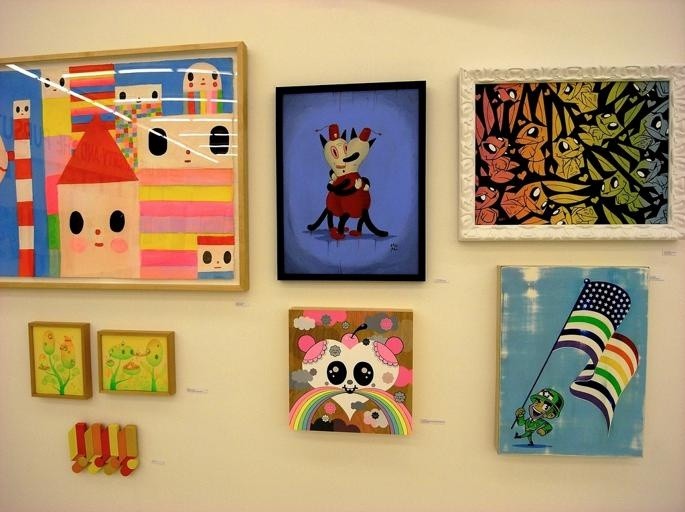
left=0, top=41, right=249, bottom=293
left=97, top=330, right=175, bottom=395
left=276, top=81, right=425, bottom=282
left=456, top=65, right=685, bottom=243
left=28, top=322, right=93, bottom=400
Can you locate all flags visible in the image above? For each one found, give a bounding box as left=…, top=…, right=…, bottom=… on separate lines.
left=553, top=276, right=641, bottom=434
